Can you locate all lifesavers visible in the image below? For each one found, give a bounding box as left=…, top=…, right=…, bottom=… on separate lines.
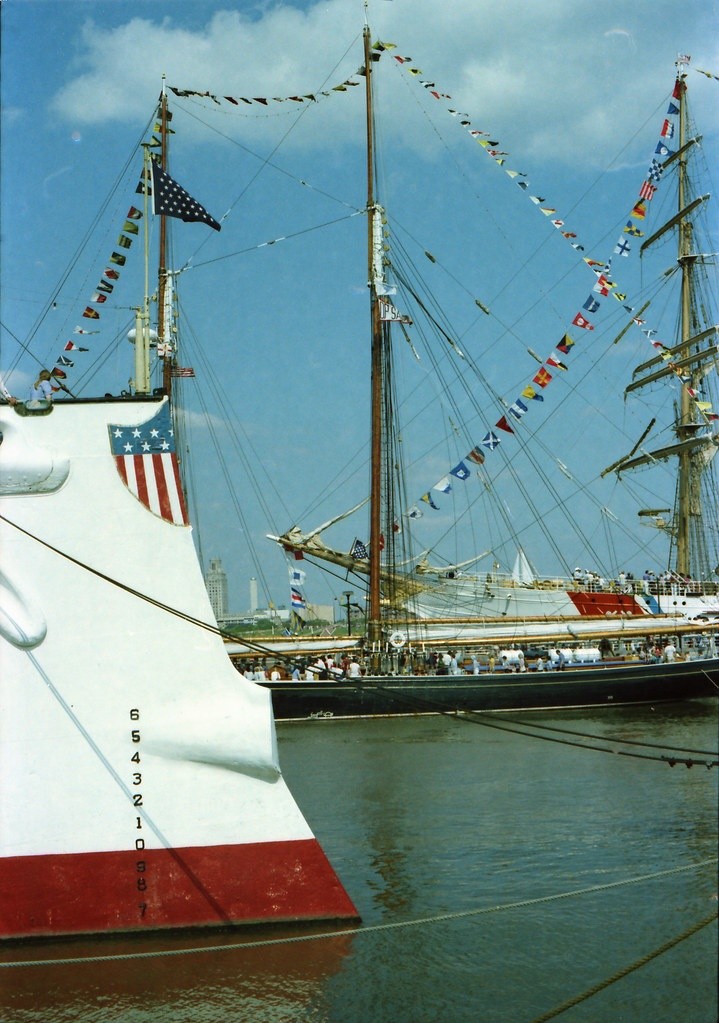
left=390, top=632, right=406, bottom=648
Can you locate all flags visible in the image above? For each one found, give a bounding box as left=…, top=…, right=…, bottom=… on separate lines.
left=288, top=565, right=306, bottom=585
left=344, top=40, right=717, bottom=560
left=283, top=543, right=303, bottom=560
left=43, top=96, right=173, bottom=394
left=290, top=587, right=307, bottom=611
left=166, top=39, right=396, bottom=105
left=147, top=151, right=221, bottom=233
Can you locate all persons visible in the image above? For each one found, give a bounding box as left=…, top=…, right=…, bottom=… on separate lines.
left=570, top=565, right=704, bottom=594
left=231, top=628, right=718, bottom=682
left=443, top=563, right=457, bottom=578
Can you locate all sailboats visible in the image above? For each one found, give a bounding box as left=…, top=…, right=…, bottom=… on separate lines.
left=137, top=1, right=719, bottom=716
left=263, top=47, right=716, bottom=622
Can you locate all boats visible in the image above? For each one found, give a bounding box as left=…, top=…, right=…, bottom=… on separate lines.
left=1, top=364, right=371, bottom=945
